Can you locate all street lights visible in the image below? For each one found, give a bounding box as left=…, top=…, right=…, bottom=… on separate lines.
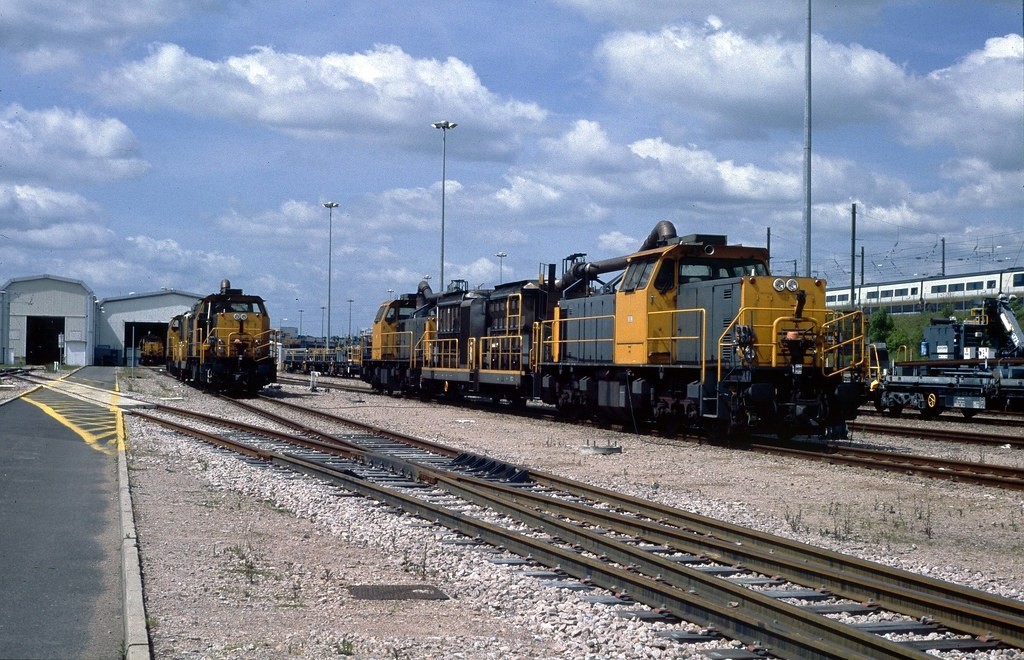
left=388, top=289, right=393, bottom=302
left=347, top=300, right=355, bottom=338
left=493, top=252, right=508, bottom=284
left=297, top=309, right=305, bottom=335
left=431, top=120, right=460, bottom=291
left=422, top=275, right=433, bottom=286
left=321, top=201, right=341, bottom=348
left=320, top=307, right=327, bottom=344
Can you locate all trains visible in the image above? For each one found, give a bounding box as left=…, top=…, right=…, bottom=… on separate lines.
left=821, top=267, right=1024, bottom=312
left=280, top=344, right=361, bottom=379
left=137, top=328, right=164, bottom=368
left=353, top=219, right=881, bottom=455
left=875, top=291, right=1024, bottom=421
left=165, top=279, right=279, bottom=397
